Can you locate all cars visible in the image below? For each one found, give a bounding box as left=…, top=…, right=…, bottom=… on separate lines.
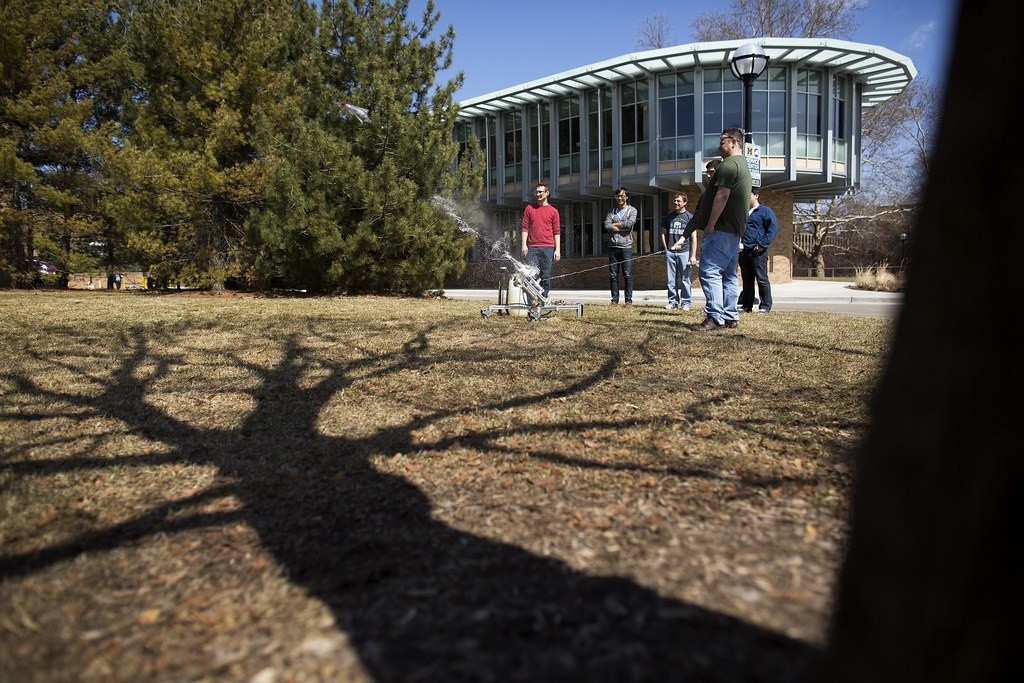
left=21, top=259, right=62, bottom=288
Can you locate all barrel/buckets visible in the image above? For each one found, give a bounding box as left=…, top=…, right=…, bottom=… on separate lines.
left=508, top=273, right=529, bottom=318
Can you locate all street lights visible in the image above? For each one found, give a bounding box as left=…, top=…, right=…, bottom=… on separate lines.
left=899, top=233, right=910, bottom=269
left=727, top=43, right=771, bottom=196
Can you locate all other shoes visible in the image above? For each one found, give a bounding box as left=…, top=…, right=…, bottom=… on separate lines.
left=725, top=319, right=738, bottom=329
left=625, top=301, right=632, bottom=306
left=611, top=301, right=618, bottom=305
left=664, top=303, right=679, bottom=310
left=737, top=306, right=752, bottom=314
left=681, top=305, right=690, bottom=311
left=690, top=317, right=720, bottom=332
left=759, top=308, right=769, bottom=315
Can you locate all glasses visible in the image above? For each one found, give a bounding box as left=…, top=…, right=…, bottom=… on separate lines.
left=705, top=170, right=715, bottom=175
left=719, top=130, right=740, bottom=143
left=615, top=194, right=627, bottom=200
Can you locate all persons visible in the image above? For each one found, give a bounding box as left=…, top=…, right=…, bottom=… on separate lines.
left=674, top=127, right=752, bottom=332
left=521, top=183, right=560, bottom=307
left=605, top=187, right=637, bottom=304
left=661, top=192, right=697, bottom=312
left=114, top=271, right=122, bottom=289
left=738, top=186, right=778, bottom=316
